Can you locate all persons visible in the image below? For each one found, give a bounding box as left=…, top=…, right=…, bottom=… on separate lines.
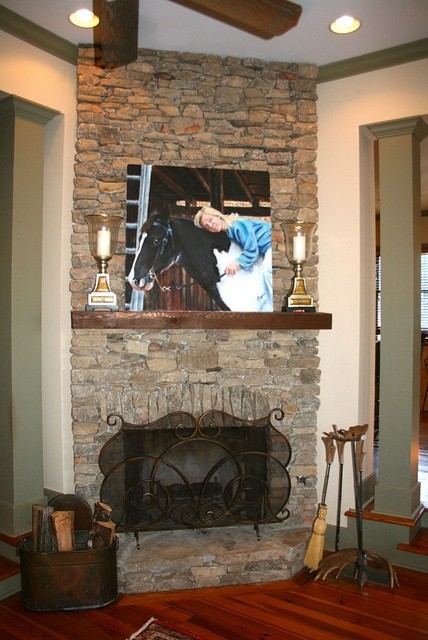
left=194, top=206, right=273, bottom=308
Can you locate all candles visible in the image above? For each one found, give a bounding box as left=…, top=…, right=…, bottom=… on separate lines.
left=94, top=225, right=111, bottom=258
left=291, top=232, right=307, bottom=260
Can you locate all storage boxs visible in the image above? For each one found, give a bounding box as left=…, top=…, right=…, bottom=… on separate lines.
left=15, top=533, right=122, bottom=615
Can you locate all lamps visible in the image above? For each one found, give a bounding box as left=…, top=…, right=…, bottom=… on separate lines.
left=83, top=213, right=125, bottom=314
left=281, top=220, right=318, bottom=310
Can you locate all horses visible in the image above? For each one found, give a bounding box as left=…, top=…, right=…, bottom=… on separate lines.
left=127, top=203, right=271, bottom=313
